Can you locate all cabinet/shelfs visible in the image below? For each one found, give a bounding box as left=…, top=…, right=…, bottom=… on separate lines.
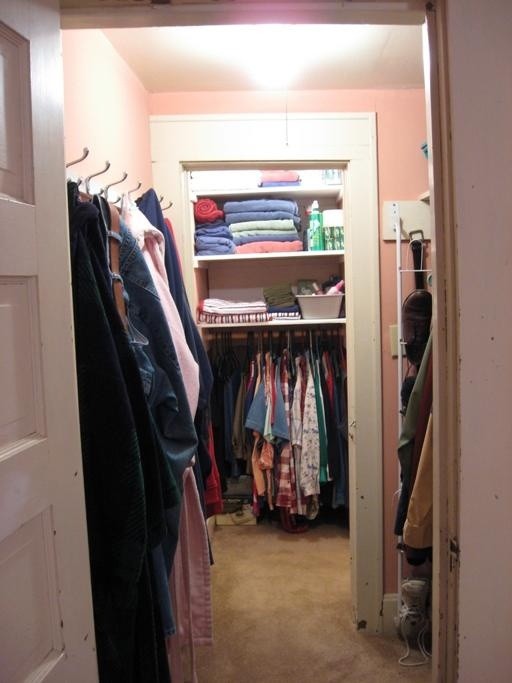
left=180, top=159, right=351, bottom=328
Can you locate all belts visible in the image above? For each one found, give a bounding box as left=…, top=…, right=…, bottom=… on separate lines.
left=107, top=201, right=128, bottom=330
left=408, top=229, right=425, bottom=289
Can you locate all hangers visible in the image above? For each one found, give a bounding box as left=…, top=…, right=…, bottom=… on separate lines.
left=206, top=327, right=347, bottom=381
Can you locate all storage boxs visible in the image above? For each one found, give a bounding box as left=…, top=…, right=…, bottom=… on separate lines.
left=295, top=294, right=346, bottom=319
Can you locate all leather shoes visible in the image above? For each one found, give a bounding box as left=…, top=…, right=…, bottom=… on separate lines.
left=401, top=290, right=432, bottom=367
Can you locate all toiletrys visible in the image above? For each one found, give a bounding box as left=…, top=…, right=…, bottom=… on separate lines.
left=312, top=282, right=323, bottom=294
left=327, top=280, right=345, bottom=294
